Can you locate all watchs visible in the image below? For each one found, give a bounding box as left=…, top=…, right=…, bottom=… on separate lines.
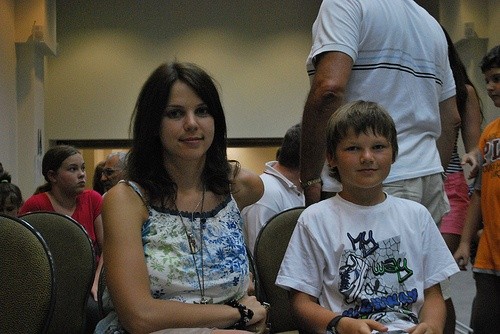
left=299, top=177, right=322, bottom=191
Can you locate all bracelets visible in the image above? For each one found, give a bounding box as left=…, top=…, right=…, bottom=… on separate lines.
left=326, top=315, right=349, bottom=334
left=225, top=300, right=254, bottom=328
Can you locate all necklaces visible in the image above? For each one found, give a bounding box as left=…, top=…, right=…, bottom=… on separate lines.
left=171, top=183, right=208, bottom=304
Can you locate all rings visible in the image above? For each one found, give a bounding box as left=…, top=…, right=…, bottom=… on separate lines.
left=262, top=302, right=270, bottom=310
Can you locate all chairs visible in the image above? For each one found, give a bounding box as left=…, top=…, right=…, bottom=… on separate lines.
left=255, top=207, right=456, bottom=334
left=18, top=211, right=97, bottom=334
left=0, top=214, right=57, bottom=334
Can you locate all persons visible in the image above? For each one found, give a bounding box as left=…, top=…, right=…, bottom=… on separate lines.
left=435, top=25, right=500, bottom=334
left=0, top=172, right=24, bottom=216
left=100, top=151, right=132, bottom=188
left=275, top=100, right=461, bottom=334
left=19, top=144, right=104, bottom=334
left=298, top=0, right=461, bottom=231
left=238, top=124, right=305, bottom=257
left=93, top=62, right=270, bottom=334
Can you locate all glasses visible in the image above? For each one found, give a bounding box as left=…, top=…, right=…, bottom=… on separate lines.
left=98, top=167, right=119, bottom=176
